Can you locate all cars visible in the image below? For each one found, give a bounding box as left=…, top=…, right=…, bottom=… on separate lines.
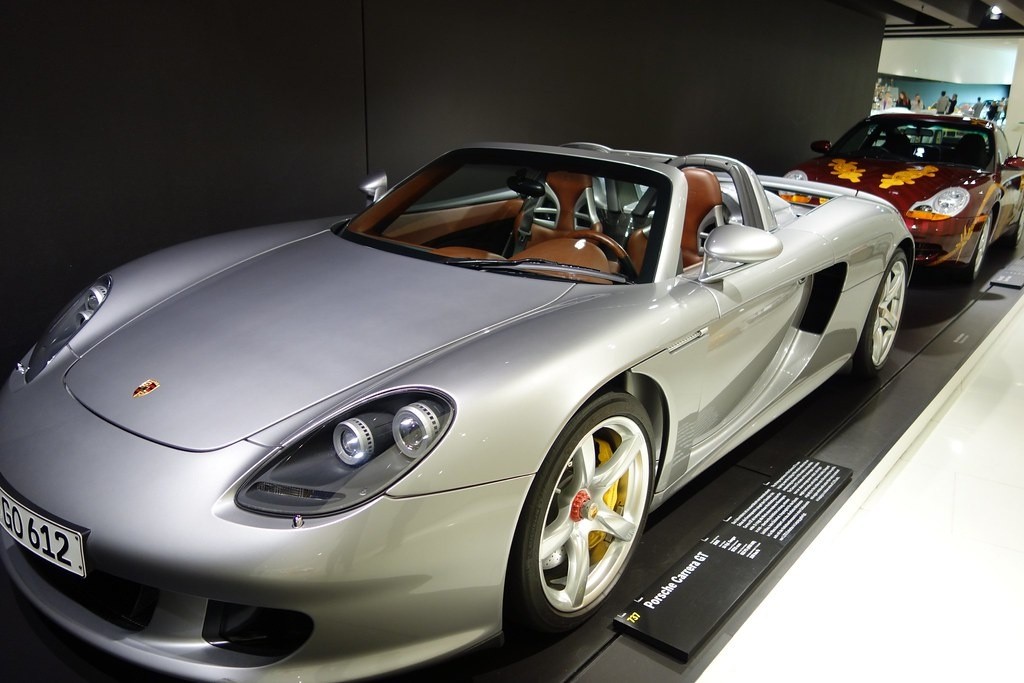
left=775, top=111, right=1024, bottom=287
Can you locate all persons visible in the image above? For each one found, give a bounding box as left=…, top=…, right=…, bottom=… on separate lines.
left=972, top=97, right=983, bottom=117
left=881, top=92, right=892, bottom=110
left=987, top=98, right=1008, bottom=127
left=937, top=91, right=957, bottom=114
left=896, top=92, right=911, bottom=110
left=911, top=93, right=923, bottom=110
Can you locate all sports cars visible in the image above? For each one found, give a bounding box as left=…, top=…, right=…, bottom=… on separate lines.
left=0, top=140, right=914, bottom=683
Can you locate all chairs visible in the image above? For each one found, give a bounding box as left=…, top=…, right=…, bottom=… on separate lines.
left=956, top=134, right=985, bottom=169
left=882, top=133, right=911, bottom=160
left=627, top=167, right=722, bottom=275
left=514, top=173, right=603, bottom=251
